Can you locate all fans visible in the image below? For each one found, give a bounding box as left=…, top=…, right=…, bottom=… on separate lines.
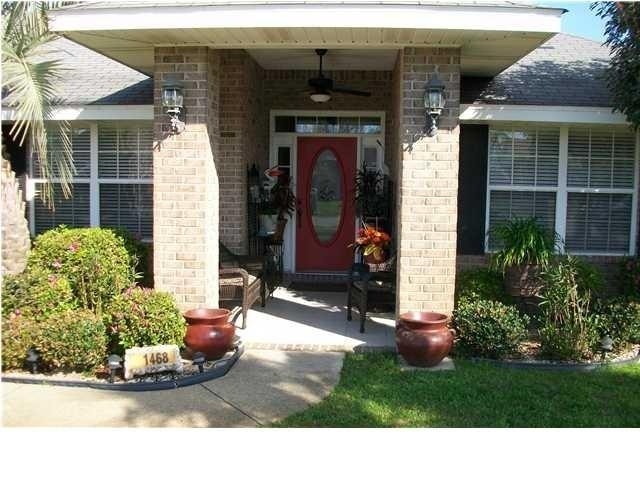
left=293, top=48, right=373, bottom=101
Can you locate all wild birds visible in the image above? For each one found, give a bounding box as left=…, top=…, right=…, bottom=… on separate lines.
left=262, top=162, right=280, bottom=202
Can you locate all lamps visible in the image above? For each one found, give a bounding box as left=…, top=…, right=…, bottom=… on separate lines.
left=160, top=70, right=188, bottom=133
left=421, top=73, right=448, bottom=137
left=310, top=91, right=332, bottom=103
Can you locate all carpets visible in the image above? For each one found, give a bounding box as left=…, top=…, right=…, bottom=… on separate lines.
left=286, top=281, right=348, bottom=293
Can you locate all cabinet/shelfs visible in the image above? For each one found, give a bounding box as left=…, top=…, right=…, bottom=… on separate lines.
left=248, top=163, right=285, bottom=258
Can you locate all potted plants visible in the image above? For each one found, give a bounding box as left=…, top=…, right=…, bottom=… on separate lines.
left=256, top=176, right=302, bottom=240
left=486, top=213, right=567, bottom=298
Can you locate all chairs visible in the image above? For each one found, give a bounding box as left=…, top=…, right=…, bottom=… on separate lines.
left=347, top=253, right=397, bottom=334
left=218, top=239, right=270, bottom=329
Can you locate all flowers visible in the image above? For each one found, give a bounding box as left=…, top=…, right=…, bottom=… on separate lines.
left=346, top=228, right=391, bottom=261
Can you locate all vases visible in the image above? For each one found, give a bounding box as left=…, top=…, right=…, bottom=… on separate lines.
left=362, top=246, right=385, bottom=263
left=182, top=307, right=236, bottom=362
left=394, top=306, right=455, bottom=370
